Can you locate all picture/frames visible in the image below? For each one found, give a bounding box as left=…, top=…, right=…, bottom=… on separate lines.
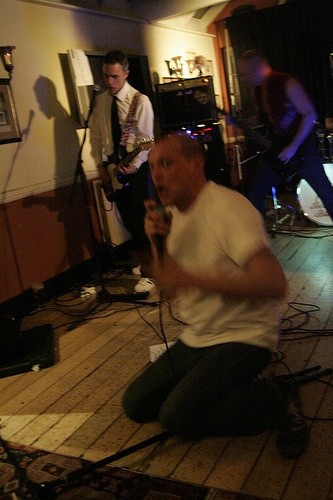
left=0, top=79, right=23, bottom=144
left=188, top=60, right=214, bottom=76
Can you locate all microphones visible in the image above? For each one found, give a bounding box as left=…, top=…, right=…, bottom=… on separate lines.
left=90, top=84, right=100, bottom=108
left=154, top=208, right=171, bottom=264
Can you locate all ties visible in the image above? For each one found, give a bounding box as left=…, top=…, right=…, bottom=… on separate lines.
left=110, top=97, right=122, bottom=154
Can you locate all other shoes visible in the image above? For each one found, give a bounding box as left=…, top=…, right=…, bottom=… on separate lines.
left=275, top=376, right=309, bottom=458
left=134, top=278, right=156, bottom=292
left=132, top=265, right=143, bottom=275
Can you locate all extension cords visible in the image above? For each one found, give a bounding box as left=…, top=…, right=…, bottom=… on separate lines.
left=80, top=286, right=96, bottom=297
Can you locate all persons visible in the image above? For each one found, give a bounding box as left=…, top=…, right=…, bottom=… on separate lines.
left=121, top=130, right=311, bottom=460
left=89, top=50, right=154, bottom=292
left=237, top=50, right=333, bottom=232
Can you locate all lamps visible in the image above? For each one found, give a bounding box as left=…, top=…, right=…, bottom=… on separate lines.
left=0, top=45, right=17, bottom=84
left=165, top=56, right=182, bottom=77
